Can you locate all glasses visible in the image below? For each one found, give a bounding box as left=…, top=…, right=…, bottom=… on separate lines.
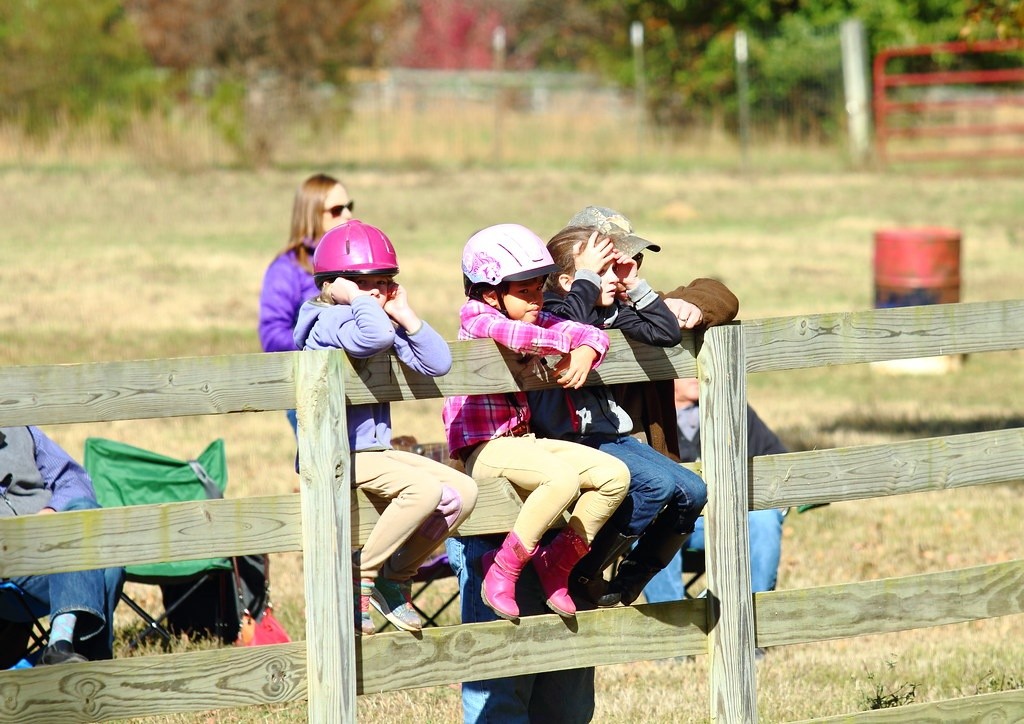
left=323, top=201, right=353, bottom=217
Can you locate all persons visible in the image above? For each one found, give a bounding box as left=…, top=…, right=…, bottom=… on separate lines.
left=443, top=205, right=738, bottom=724
left=295, top=220, right=477, bottom=635
left=1, top=425, right=125, bottom=665
left=643, top=378, right=789, bottom=603
left=258, top=175, right=355, bottom=432
left=526, top=226, right=707, bottom=608
left=442, top=223, right=630, bottom=620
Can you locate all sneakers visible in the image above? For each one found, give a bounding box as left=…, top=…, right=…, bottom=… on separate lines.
left=352, top=550, right=376, bottom=636
left=369, top=564, right=422, bottom=632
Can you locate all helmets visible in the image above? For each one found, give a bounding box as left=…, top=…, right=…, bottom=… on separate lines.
left=313, top=220, right=399, bottom=291
left=461, top=224, right=563, bottom=297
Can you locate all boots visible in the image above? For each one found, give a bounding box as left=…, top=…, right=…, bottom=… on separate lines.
left=608, top=511, right=695, bottom=606
left=568, top=526, right=646, bottom=607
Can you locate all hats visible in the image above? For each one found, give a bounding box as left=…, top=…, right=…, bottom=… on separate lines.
left=567, top=205, right=661, bottom=259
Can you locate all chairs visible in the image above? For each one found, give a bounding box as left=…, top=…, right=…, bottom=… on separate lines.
left=84, top=436, right=242, bottom=645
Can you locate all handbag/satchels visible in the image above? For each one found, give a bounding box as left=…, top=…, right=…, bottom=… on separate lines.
left=188, top=448, right=290, bottom=646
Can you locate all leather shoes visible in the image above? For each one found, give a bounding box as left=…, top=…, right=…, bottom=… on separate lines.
left=35, top=639, right=89, bottom=668
left=475, top=532, right=540, bottom=621
left=531, top=524, right=591, bottom=618
